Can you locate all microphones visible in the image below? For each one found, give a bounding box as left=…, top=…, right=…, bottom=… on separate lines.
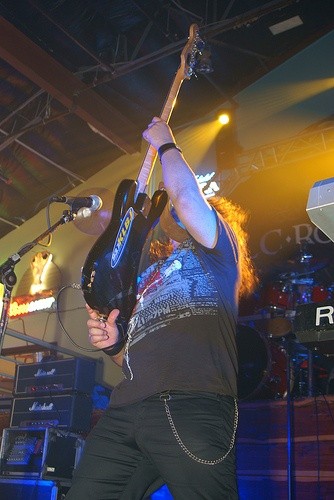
left=53, top=195, right=102, bottom=212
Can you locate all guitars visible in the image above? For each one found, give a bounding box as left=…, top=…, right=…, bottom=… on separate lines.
left=79, top=22, right=207, bottom=323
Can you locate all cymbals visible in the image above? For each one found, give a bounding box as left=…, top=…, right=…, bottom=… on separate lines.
left=283, top=242, right=330, bottom=273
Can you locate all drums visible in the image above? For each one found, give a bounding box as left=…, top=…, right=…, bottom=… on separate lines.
left=279, top=263, right=334, bottom=306
left=258, top=280, right=292, bottom=313
left=254, top=310, right=292, bottom=337
left=237, top=323, right=294, bottom=403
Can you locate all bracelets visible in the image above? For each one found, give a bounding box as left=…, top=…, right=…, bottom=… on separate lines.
left=103, top=333, right=125, bottom=356
left=156, top=142, right=184, bottom=164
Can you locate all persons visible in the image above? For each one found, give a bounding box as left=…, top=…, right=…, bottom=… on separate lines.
left=64, top=117, right=257, bottom=500
